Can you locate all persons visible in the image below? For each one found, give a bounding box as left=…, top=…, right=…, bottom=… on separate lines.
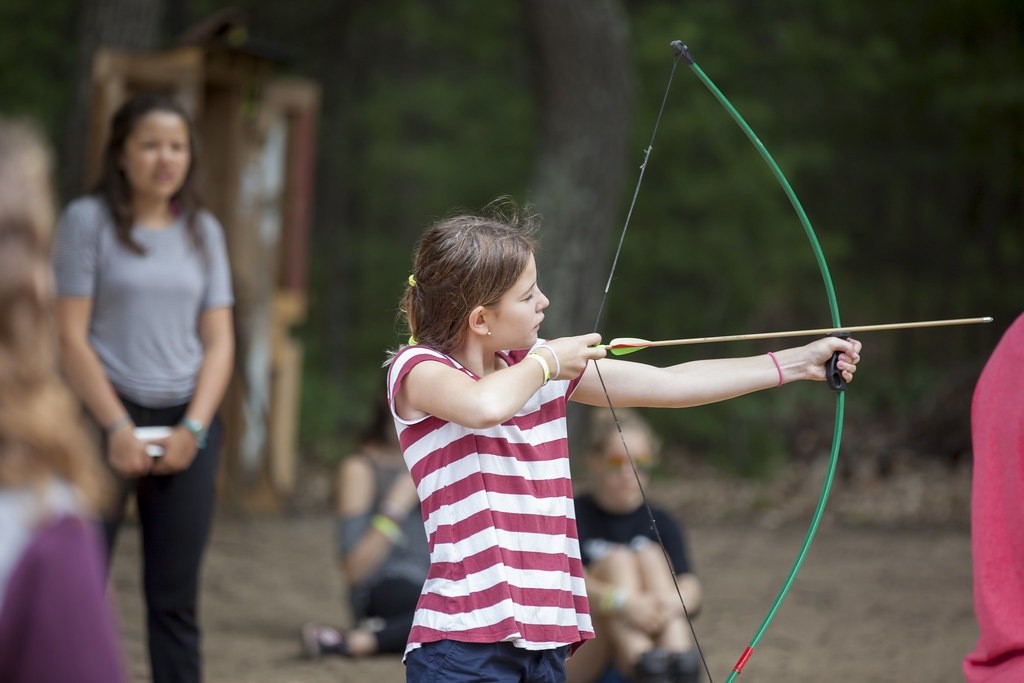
left=300, top=384, right=430, bottom=659
left=0, top=140, right=127, bottom=683
left=565, top=406, right=705, bottom=683
left=50, top=102, right=235, bottom=683
left=387, top=217, right=862, bottom=683
left=963, top=315, right=1024, bottom=683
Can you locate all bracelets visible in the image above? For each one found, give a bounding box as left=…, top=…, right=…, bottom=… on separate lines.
left=181, top=418, right=207, bottom=448
left=372, top=503, right=408, bottom=541
left=525, top=345, right=560, bottom=386
left=599, top=586, right=633, bottom=616
left=768, top=352, right=784, bottom=387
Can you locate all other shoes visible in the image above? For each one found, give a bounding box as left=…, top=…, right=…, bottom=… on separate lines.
left=299, top=622, right=350, bottom=659
left=626, top=650, right=700, bottom=683
